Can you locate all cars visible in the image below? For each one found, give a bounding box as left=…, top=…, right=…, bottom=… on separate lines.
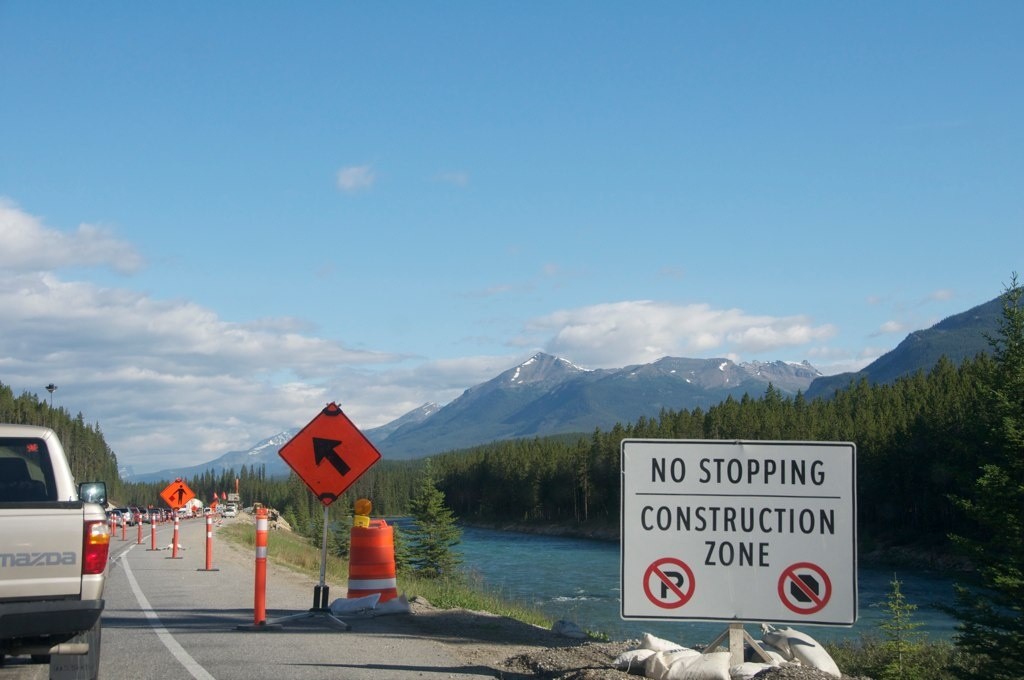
left=204, top=503, right=243, bottom=518
left=105, top=507, right=203, bottom=528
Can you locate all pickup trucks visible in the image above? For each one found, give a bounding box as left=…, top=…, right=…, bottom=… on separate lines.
left=0, top=424, right=111, bottom=680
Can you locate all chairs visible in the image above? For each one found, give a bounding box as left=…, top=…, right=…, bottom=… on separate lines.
left=0, top=457, right=45, bottom=501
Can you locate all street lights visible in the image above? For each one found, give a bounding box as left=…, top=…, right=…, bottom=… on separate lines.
left=46, top=383, right=58, bottom=429
